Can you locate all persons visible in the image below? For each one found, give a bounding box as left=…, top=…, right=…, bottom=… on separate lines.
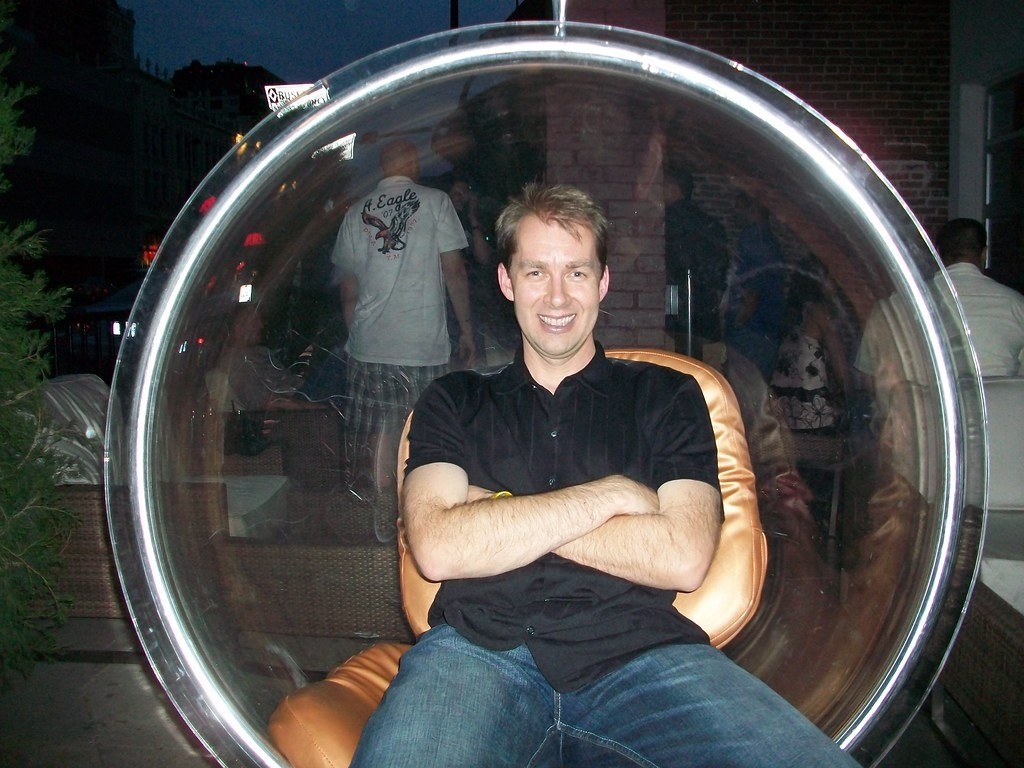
left=226, top=307, right=346, bottom=410
left=852, top=218, right=1024, bottom=419
left=729, top=191, right=782, bottom=348
left=333, top=140, right=496, bottom=491
left=350, top=184, right=860, bottom=767
left=664, top=167, right=729, bottom=336
left=769, top=257, right=864, bottom=438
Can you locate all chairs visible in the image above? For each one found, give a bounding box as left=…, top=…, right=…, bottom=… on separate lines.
left=64, top=308, right=145, bottom=426
left=826, top=373, right=1024, bottom=570
left=261, top=349, right=770, bottom=768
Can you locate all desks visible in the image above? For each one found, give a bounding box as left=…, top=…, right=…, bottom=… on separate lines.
left=42, top=274, right=171, bottom=400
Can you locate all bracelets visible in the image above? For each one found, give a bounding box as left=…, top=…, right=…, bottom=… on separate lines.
left=458, top=318, right=472, bottom=323
left=472, top=225, right=481, bottom=229
left=490, top=491, right=512, bottom=498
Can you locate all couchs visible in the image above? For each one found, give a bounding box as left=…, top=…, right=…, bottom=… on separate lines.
left=217, top=394, right=416, bottom=672
left=913, top=500, right=1024, bottom=768
left=790, top=427, right=849, bottom=471
left=4, top=373, right=286, bottom=656
left=207, top=361, right=342, bottom=491
